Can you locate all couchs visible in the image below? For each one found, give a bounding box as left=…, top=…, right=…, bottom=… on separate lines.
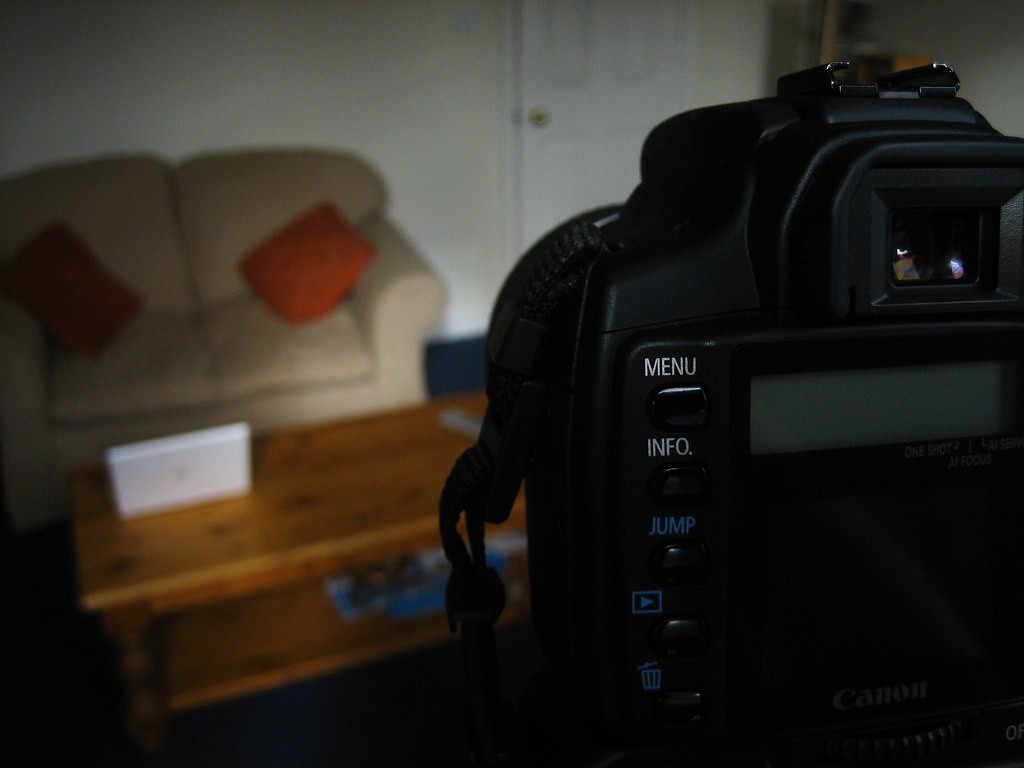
left=0, top=148, right=450, bottom=530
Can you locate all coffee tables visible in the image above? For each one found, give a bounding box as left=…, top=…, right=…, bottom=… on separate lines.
left=70, top=392, right=534, bottom=751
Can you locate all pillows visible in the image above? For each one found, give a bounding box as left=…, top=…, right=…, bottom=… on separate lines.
left=0, top=216, right=147, bottom=367
left=231, top=207, right=372, bottom=326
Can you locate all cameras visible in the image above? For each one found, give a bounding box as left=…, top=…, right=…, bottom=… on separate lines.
left=505, top=57, right=1024, bottom=768
